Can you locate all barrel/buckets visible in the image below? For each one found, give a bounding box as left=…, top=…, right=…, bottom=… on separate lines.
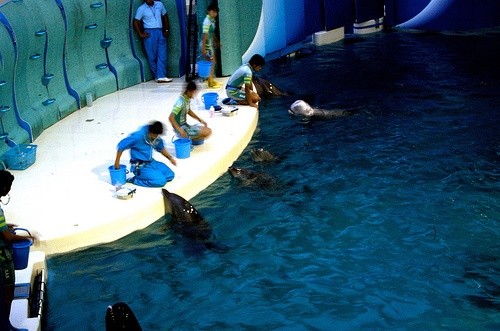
left=9, top=228, right=34, bottom=270
left=171, top=132, right=192, bottom=159
left=201, top=93, right=219, bottom=111
left=197, top=55, right=213, bottom=78
left=108, top=164, right=129, bottom=185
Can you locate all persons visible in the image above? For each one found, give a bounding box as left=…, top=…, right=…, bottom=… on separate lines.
left=221, top=53, right=265, bottom=109
left=198, top=3, right=223, bottom=89
left=169, top=82, right=212, bottom=146
left=132, top=0, right=173, bottom=83
left=0, top=170, right=37, bottom=331
left=115, top=121, right=177, bottom=187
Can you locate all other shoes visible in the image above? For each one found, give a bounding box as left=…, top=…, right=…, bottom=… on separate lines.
left=222, top=97, right=236, bottom=105
left=208, top=81, right=223, bottom=88
left=156, top=77, right=173, bottom=83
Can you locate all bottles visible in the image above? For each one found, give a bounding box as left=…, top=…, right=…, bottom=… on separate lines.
left=209, top=106, right=215, bottom=118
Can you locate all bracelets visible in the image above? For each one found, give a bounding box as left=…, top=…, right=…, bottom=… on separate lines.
left=164, top=29, right=169, bottom=32
left=167, top=155, right=171, bottom=159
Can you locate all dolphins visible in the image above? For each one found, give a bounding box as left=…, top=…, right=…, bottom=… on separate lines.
left=228, top=167, right=251, bottom=182
left=104, top=302, right=143, bottom=331
left=162, top=188, right=211, bottom=239
left=287, top=100, right=351, bottom=118
left=252, top=75, right=287, bottom=97
left=250, top=147, right=274, bottom=161
